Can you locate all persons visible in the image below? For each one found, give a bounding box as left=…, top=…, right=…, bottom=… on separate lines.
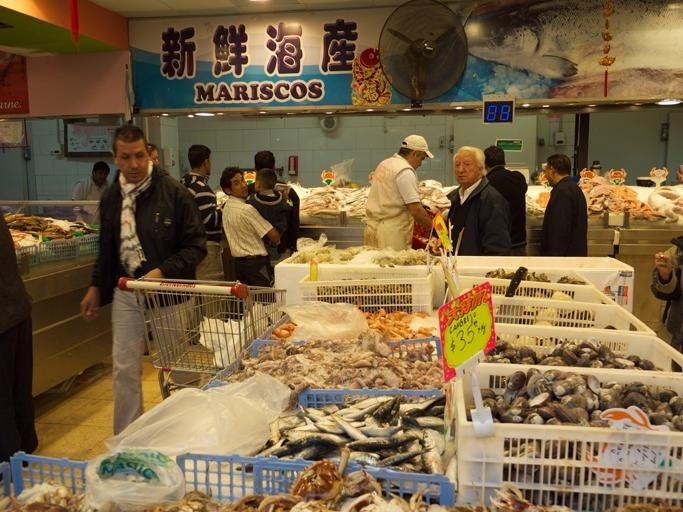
left=538, top=153, right=588, bottom=257
left=483, top=145, right=528, bottom=256
left=145, top=142, right=300, bottom=322
left=649, top=235, right=682, bottom=372
left=428, top=145, right=514, bottom=256
left=362, top=134, right=434, bottom=252
left=79, top=126, right=209, bottom=436
left=0, top=208, right=39, bottom=470
left=70, top=160, right=112, bottom=225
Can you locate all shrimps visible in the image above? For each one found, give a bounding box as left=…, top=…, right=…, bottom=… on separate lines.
left=270, top=308, right=436, bottom=341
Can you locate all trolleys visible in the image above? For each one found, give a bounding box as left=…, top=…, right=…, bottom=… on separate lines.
left=116, top=276, right=286, bottom=399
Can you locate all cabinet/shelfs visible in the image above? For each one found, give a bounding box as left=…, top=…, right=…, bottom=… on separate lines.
left=526, top=226, right=682, bottom=326
left=20, top=254, right=112, bottom=398
left=298, top=225, right=364, bottom=249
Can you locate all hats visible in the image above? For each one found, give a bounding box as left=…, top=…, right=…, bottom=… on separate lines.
left=399, top=134, right=435, bottom=160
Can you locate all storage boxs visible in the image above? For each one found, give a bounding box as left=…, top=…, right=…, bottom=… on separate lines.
left=453, top=255, right=633, bottom=330
left=273, top=252, right=444, bottom=310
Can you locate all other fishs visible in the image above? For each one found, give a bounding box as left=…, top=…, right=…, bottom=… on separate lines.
left=236, top=395, right=445, bottom=489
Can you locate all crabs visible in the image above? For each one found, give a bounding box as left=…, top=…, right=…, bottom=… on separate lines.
left=10, top=448, right=573, bottom=512
left=606, top=502, right=683, bottom=512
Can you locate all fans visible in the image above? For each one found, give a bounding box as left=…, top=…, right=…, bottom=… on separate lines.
left=378, top=1, right=466, bottom=103
